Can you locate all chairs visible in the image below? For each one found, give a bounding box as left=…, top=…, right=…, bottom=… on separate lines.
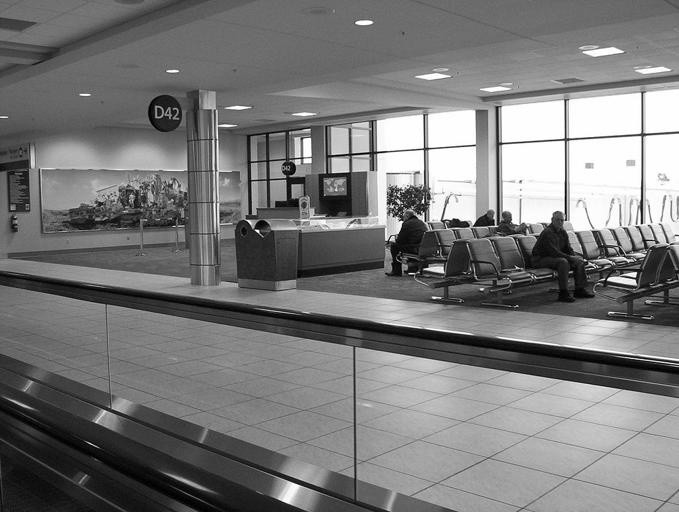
left=395, top=219, right=678, bottom=322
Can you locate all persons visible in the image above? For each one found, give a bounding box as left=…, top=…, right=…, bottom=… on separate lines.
left=385, top=210, right=430, bottom=277
left=498, top=211, right=525, bottom=234
left=529, top=211, right=595, bottom=302
left=473, top=209, right=495, bottom=226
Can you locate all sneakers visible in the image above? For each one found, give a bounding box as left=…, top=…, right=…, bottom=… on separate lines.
left=403, top=268, right=416, bottom=272
left=385, top=271, right=401, bottom=276
left=559, top=290, right=575, bottom=302
left=574, top=288, right=594, bottom=298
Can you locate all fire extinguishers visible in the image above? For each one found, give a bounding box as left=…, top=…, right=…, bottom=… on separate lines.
left=11, top=213, right=19, bottom=229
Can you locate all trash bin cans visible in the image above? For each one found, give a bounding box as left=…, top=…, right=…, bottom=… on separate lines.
left=235, top=218, right=299, bottom=291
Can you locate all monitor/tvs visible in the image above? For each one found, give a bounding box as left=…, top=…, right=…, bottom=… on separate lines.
left=319, top=173, right=352, bottom=200
